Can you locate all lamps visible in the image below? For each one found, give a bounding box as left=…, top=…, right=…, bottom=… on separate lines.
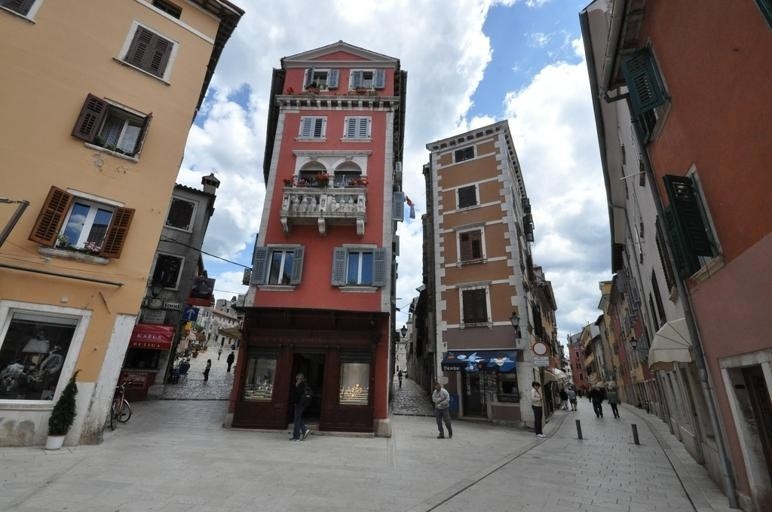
left=509, top=310, right=522, bottom=339
left=144, top=281, right=163, bottom=300
left=629, top=336, right=648, bottom=352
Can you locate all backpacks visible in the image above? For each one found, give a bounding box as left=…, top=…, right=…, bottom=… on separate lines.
left=304, top=383, right=313, bottom=407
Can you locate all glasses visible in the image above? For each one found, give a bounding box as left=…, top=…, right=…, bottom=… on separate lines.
left=297, top=377, right=303, bottom=380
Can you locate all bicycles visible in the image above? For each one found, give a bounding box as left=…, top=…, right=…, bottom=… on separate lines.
left=109, top=381, right=132, bottom=430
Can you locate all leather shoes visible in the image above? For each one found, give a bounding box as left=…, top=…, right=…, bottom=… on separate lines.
left=449, top=432, right=452, bottom=437
left=437, top=435, right=444, bottom=438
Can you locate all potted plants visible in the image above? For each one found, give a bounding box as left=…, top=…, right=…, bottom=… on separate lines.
left=43, top=368, right=81, bottom=451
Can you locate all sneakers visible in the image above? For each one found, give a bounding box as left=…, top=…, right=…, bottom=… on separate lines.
left=290, top=437, right=300, bottom=441
left=536, top=433, right=543, bottom=437
left=301, top=429, right=311, bottom=439
left=542, top=433, right=546, bottom=437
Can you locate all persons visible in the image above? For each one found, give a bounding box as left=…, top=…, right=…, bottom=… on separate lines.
left=288, top=373, right=313, bottom=441
left=216, top=346, right=223, bottom=360
left=431, top=382, right=453, bottom=438
left=201, top=358, right=211, bottom=381
left=225, top=350, right=235, bottom=372
left=396, top=369, right=403, bottom=388
left=559, top=382, right=622, bottom=419
left=528, top=380, right=548, bottom=438
